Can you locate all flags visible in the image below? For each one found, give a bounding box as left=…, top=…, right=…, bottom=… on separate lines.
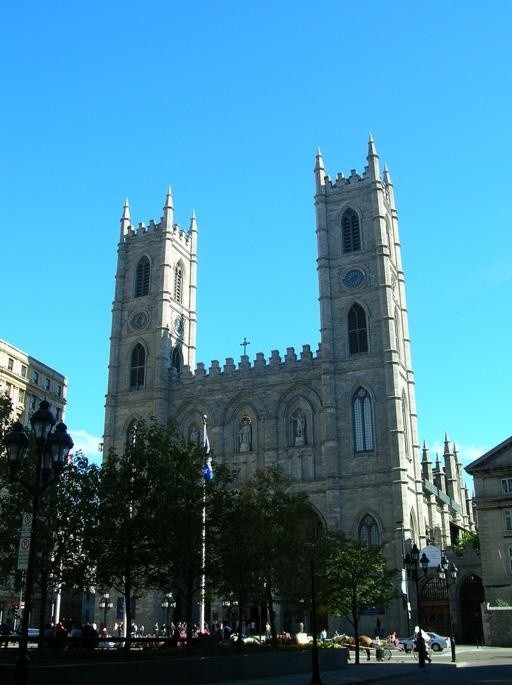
left=203, top=435, right=215, bottom=482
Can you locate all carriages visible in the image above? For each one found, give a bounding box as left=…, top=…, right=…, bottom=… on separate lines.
left=331, top=632, right=404, bottom=662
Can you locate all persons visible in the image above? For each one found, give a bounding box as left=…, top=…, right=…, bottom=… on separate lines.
left=58, top=621, right=232, bottom=652
left=332, top=630, right=339, bottom=637
left=238, top=419, right=252, bottom=453
left=190, top=425, right=199, bottom=448
left=265, top=622, right=271, bottom=639
left=321, top=628, right=328, bottom=642
left=0, top=622, right=10, bottom=649
left=390, top=632, right=397, bottom=645
left=413, top=632, right=432, bottom=664
left=289, top=413, right=306, bottom=438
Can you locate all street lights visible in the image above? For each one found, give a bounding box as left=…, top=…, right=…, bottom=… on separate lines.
left=223, top=590, right=239, bottom=629
left=301, top=542, right=325, bottom=683
left=161, top=591, right=177, bottom=638
left=404, top=543, right=431, bottom=668
left=438, top=554, right=460, bottom=663
left=99, top=593, right=114, bottom=628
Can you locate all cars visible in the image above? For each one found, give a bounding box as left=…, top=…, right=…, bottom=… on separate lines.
left=28, top=628, right=40, bottom=640
left=398, top=631, right=455, bottom=653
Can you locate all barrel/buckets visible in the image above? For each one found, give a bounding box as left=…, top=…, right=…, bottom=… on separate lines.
left=375, top=650, right=385, bottom=661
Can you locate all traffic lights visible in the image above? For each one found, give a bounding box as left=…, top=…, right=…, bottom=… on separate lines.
left=13, top=606, right=19, bottom=616
